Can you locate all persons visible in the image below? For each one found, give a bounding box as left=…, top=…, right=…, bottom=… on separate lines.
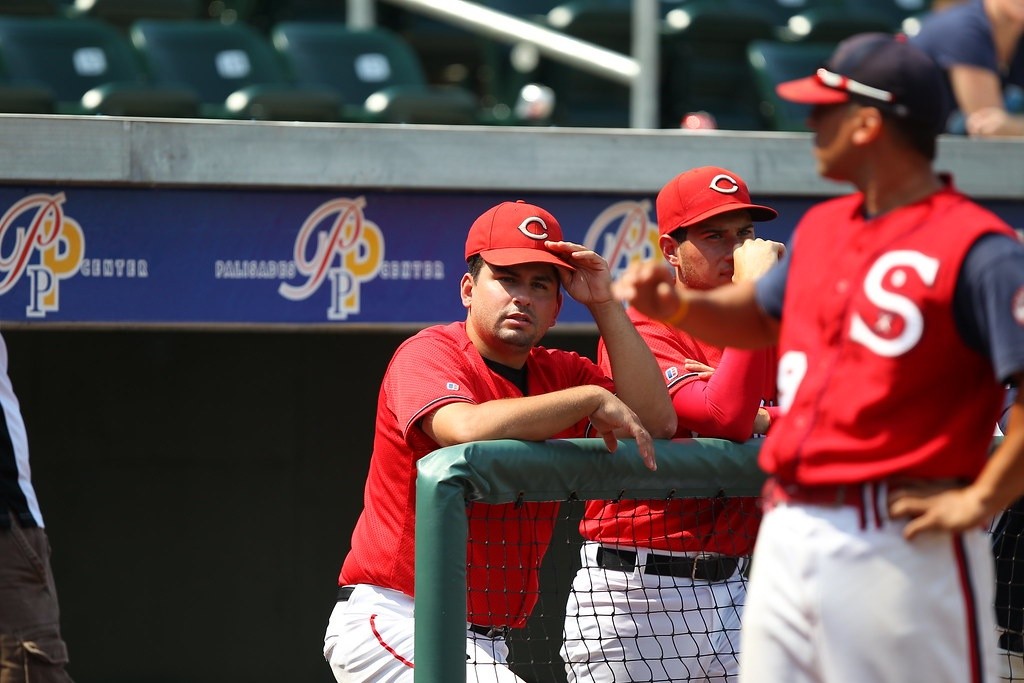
left=0, top=333, right=71, bottom=681
left=321, top=199, right=677, bottom=683
left=607, top=32, right=1024, bottom=681
left=915, top=0, right=1024, bottom=134
left=562, top=167, right=778, bottom=681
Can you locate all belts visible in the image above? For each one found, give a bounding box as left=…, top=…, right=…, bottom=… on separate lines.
left=765, top=480, right=860, bottom=504
left=596, top=548, right=736, bottom=579
left=336, top=588, right=508, bottom=640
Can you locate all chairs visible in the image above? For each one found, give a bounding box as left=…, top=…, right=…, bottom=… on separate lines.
left=0, top=1, right=944, bottom=135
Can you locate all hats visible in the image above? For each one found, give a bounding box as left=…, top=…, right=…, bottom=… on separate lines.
left=464, top=199, right=575, bottom=269
left=775, top=32, right=950, bottom=135
left=657, top=166, right=778, bottom=237
